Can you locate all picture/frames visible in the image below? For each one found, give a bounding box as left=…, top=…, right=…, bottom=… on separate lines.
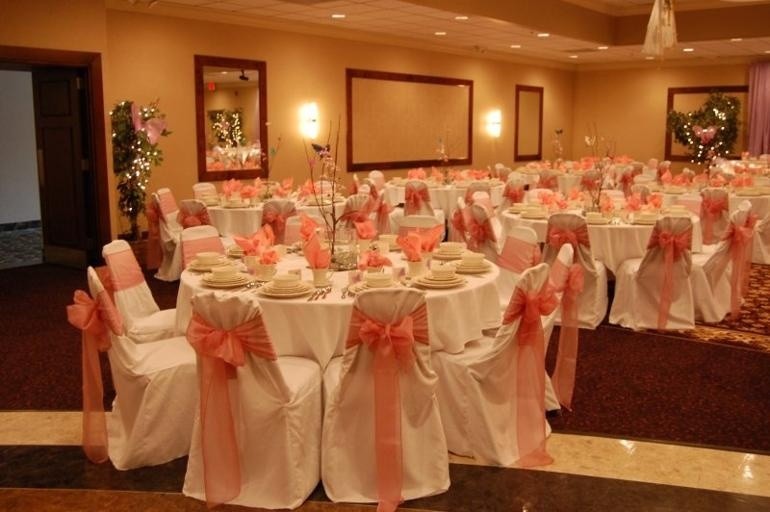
left=346, top=69, right=473, bottom=172
left=514, top=84, right=543, bottom=162
left=662, top=84, right=750, bottom=162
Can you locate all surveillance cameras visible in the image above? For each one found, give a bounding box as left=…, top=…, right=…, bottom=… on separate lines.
left=239, top=75, right=249, bottom=80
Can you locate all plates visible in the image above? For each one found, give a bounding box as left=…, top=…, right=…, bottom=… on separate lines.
left=647, top=184, right=770, bottom=196
left=190, top=260, right=231, bottom=271
left=197, top=192, right=342, bottom=210
left=350, top=281, right=404, bottom=296
left=200, top=276, right=253, bottom=287
left=413, top=274, right=467, bottom=289
left=258, top=285, right=313, bottom=296
left=449, top=260, right=494, bottom=274
left=508, top=203, right=693, bottom=227
left=432, top=252, right=466, bottom=260
left=391, top=178, right=502, bottom=188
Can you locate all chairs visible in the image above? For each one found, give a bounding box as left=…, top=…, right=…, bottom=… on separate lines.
left=180, top=289, right=326, bottom=511
left=84, top=264, right=198, bottom=474
left=319, top=285, right=454, bottom=506
left=427, top=259, right=554, bottom=470
left=100, top=234, right=180, bottom=342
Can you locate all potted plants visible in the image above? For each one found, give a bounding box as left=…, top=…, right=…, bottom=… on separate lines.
left=109, top=98, right=174, bottom=271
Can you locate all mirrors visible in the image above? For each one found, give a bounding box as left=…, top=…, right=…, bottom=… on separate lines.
left=193, top=55, right=269, bottom=179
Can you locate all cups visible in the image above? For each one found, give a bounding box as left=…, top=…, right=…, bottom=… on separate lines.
left=213, top=267, right=238, bottom=279
left=228, top=248, right=244, bottom=256
left=243, top=256, right=258, bottom=274
left=440, top=243, right=466, bottom=254
left=365, top=272, right=391, bottom=287
left=197, top=253, right=219, bottom=264
left=313, top=267, right=333, bottom=287
left=431, top=265, right=456, bottom=279
left=272, top=274, right=301, bottom=288
left=256, top=265, right=278, bottom=281
left=464, top=253, right=485, bottom=265
